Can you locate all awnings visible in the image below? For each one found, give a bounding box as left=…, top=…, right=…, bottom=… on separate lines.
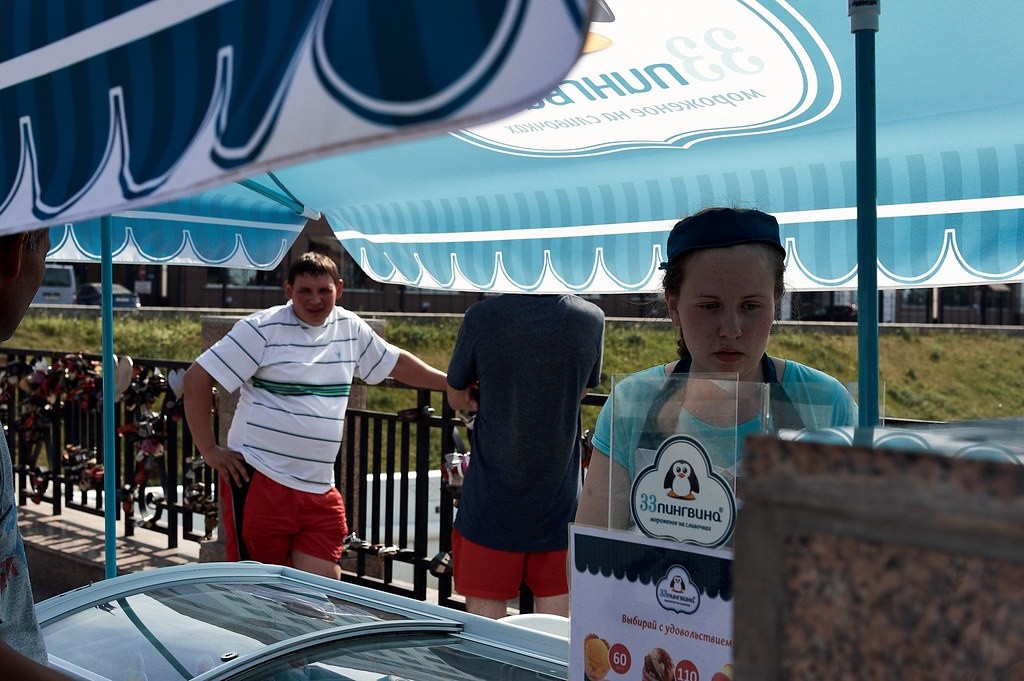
left=0, top=0, right=1024, bottom=576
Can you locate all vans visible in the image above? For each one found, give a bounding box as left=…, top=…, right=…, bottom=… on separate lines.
left=31, top=264, right=77, bottom=304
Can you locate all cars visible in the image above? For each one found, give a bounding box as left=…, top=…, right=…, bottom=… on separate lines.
left=77, top=282, right=141, bottom=306
left=796, top=303, right=859, bottom=321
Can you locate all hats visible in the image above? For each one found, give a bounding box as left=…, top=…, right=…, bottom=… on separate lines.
left=659, top=208, right=780, bottom=271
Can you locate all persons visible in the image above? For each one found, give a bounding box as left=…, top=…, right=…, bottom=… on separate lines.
left=447, top=293, right=605, bottom=621
left=574, top=208, right=859, bottom=552
left=0, top=227, right=79, bottom=681
left=183, top=252, right=479, bottom=581
